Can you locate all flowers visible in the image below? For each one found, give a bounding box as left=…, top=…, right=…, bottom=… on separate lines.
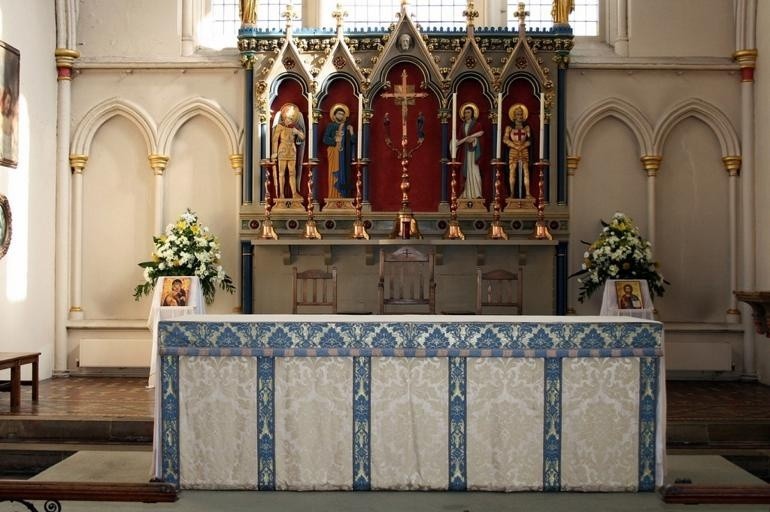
left=130, top=207, right=237, bottom=307
left=566, top=212, right=671, bottom=305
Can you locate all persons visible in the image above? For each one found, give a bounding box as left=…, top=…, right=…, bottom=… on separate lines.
left=1, top=86, right=19, bottom=161
left=270, top=103, right=306, bottom=200
left=321, top=103, right=358, bottom=198
left=620, top=284, right=643, bottom=309
left=163, top=279, right=187, bottom=306
left=502, top=103, right=535, bottom=199
left=456, top=101, right=485, bottom=199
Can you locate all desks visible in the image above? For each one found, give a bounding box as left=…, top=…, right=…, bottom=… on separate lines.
left=156, top=314, right=665, bottom=493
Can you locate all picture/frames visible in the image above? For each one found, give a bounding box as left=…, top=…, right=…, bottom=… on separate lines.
left=0, top=38, right=22, bottom=170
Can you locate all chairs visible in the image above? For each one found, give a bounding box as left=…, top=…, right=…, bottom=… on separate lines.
left=291, top=249, right=523, bottom=315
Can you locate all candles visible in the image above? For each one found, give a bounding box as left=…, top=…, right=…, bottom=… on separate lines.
left=266, top=94, right=363, bottom=159
left=452, top=90, right=545, bottom=161
